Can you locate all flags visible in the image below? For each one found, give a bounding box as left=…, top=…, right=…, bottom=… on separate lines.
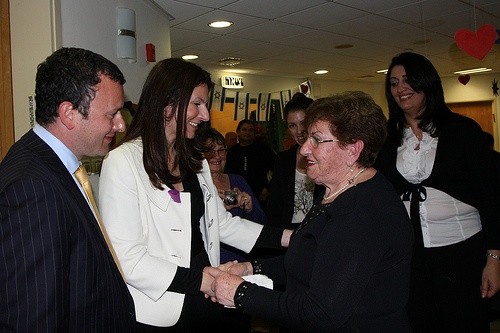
left=207, top=83, right=225, bottom=112
left=279, top=90, right=292, bottom=119
left=298, top=80, right=312, bottom=98
left=233, top=91, right=250, bottom=122
left=255, top=93, right=271, bottom=122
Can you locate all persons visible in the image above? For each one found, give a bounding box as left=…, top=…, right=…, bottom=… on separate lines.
left=99, top=57, right=294, bottom=332
left=371, top=51, right=500, bottom=333
left=0, top=47, right=137, bottom=333
left=194, top=127, right=268, bottom=333
left=223, top=119, right=276, bottom=216
left=263, top=92, right=325, bottom=253
left=205, top=90, right=437, bottom=333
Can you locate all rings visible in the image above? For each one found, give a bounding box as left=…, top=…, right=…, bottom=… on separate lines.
left=244, top=196, right=247, bottom=200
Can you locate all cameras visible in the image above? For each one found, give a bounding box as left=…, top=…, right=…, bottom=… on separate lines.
left=222, top=190, right=238, bottom=205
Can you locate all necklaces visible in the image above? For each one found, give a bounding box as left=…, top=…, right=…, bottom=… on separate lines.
left=416, top=135, right=419, bottom=138
left=323, top=169, right=365, bottom=200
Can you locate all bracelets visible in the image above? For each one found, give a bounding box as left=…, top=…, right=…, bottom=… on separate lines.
left=485, top=252, right=500, bottom=264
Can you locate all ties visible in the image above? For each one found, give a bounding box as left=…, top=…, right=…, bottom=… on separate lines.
left=75, top=165, right=126, bottom=284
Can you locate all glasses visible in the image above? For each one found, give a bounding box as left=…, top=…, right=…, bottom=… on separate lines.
left=302, top=131, right=354, bottom=149
left=203, top=147, right=229, bottom=161
